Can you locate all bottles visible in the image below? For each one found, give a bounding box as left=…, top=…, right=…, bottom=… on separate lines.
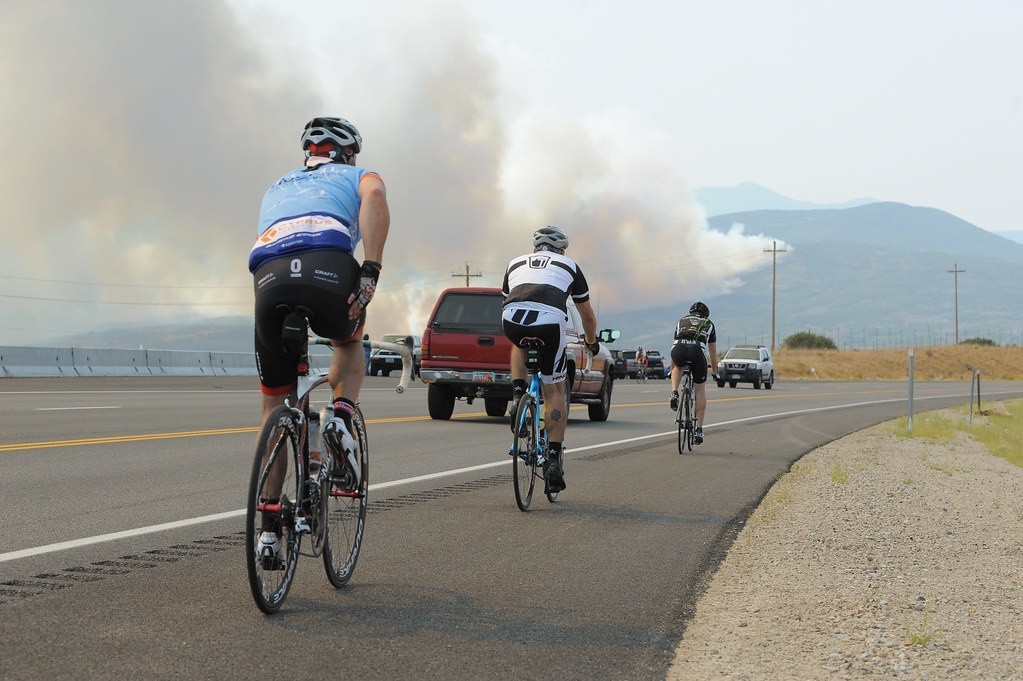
left=309, top=411, right=321, bottom=463
left=318, top=406, right=335, bottom=462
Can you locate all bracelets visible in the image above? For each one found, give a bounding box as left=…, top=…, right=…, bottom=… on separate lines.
left=363, top=260, right=382, bottom=270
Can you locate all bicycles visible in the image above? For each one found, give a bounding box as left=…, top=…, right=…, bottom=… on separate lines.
left=511, top=343, right=595, bottom=513
left=243, top=336, right=416, bottom=616
left=636, top=365, right=649, bottom=384
left=677, top=360, right=701, bottom=457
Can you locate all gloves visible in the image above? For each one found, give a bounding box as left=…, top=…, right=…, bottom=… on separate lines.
left=711, top=373, right=721, bottom=381
left=584, top=336, right=600, bottom=357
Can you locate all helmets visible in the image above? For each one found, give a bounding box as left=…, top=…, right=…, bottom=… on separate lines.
left=301, top=116, right=362, bottom=166
left=689, top=302, right=710, bottom=317
left=532, top=225, right=569, bottom=255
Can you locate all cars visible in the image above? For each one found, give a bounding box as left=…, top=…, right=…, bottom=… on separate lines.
left=716, top=344, right=777, bottom=391
left=369, top=334, right=421, bottom=376
left=417, top=287, right=620, bottom=421
left=604, top=349, right=666, bottom=381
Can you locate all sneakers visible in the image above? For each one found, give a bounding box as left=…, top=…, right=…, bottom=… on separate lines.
left=256, top=532, right=286, bottom=561
left=323, top=417, right=362, bottom=493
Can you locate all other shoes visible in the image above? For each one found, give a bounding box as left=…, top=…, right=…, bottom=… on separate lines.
left=509, top=403, right=528, bottom=439
left=544, top=458, right=567, bottom=491
left=669, top=391, right=679, bottom=411
left=694, top=427, right=704, bottom=443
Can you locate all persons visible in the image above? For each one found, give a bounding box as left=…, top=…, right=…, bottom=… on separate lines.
left=635, top=346, right=649, bottom=380
left=502, top=225, right=600, bottom=491
left=668, top=302, right=719, bottom=443
left=248, top=118, right=390, bottom=570
left=361, top=334, right=371, bottom=376
